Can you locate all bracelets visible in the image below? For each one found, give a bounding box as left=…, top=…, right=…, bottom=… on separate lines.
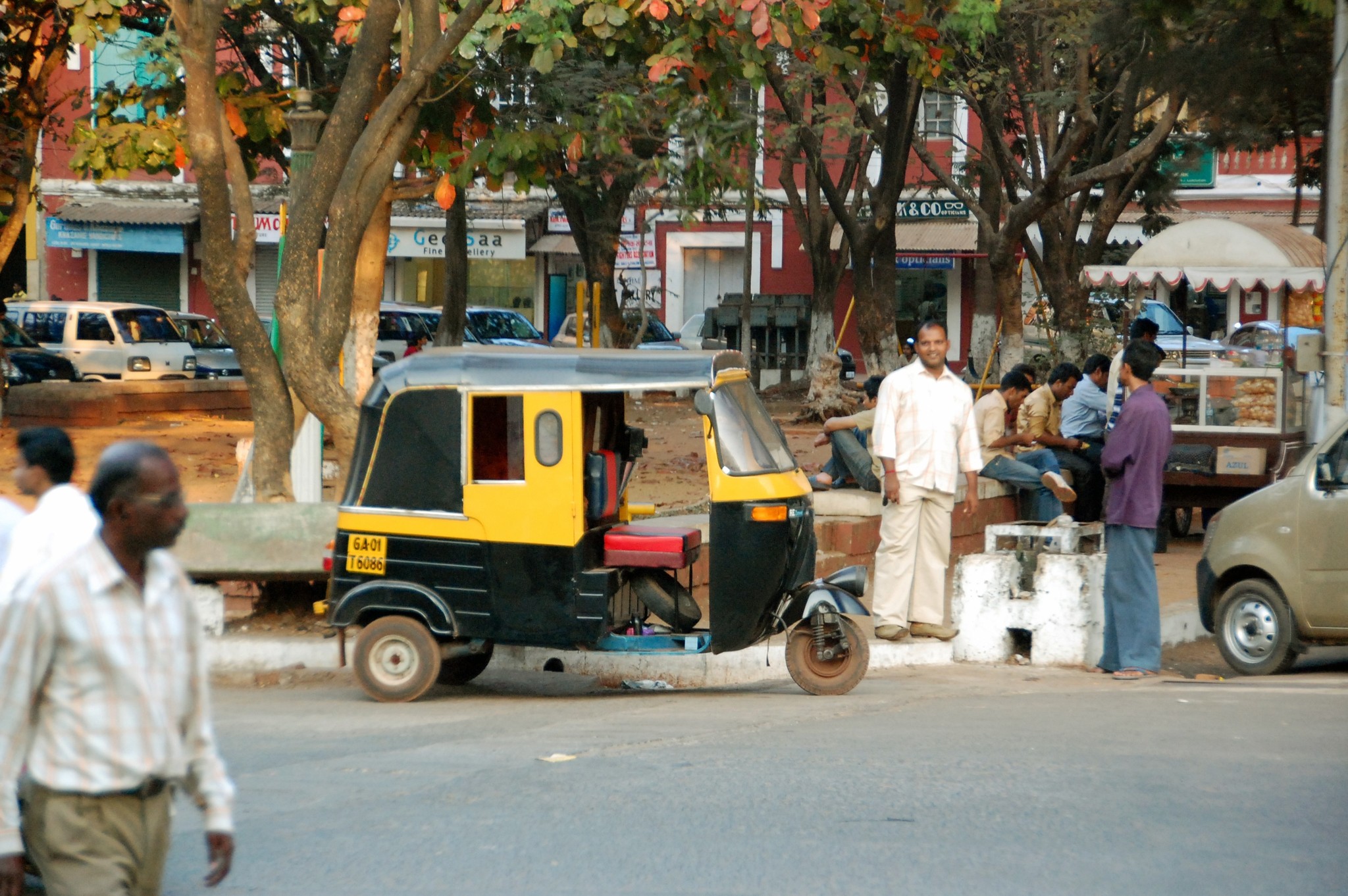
left=885, top=470, right=897, bottom=474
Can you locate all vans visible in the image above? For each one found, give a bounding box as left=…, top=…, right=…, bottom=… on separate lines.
left=3, top=299, right=198, bottom=383
left=164, top=310, right=246, bottom=381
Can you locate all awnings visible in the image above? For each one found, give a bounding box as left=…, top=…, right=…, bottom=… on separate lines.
left=828, top=223, right=978, bottom=251
left=1022, top=223, right=1155, bottom=247
left=528, top=235, right=581, bottom=255
left=56, top=189, right=551, bottom=224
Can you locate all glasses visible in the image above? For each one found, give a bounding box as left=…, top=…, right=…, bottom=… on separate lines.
left=128, top=490, right=184, bottom=510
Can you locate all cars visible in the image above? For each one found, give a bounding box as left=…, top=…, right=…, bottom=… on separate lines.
left=372, top=301, right=482, bottom=378
left=430, top=303, right=557, bottom=347
left=1219, top=320, right=1285, bottom=350
left=1194, top=417, right=1348, bottom=677
left=1020, top=290, right=1227, bottom=370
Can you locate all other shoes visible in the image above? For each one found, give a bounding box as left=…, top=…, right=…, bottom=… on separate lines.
left=1041, top=471, right=1077, bottom=503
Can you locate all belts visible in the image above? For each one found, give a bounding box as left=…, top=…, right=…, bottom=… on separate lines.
left=124, top=776, right=165, bottom=800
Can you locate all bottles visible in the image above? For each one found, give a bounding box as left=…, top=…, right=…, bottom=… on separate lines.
left=1043, top=515, right=1074, bottom=553
left=1256, top=334, right=1283, bottom=350
left=1205, top=394, right=1214, bottom=425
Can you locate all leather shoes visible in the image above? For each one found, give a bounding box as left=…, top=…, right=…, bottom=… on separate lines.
left=909, top=622, right=959, bottom=641
left=874, top=624, right=909, bottom=641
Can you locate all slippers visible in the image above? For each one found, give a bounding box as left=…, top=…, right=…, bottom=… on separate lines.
left=807, top=475, right=829, bottom=491
left=832, top=477, right=860, bottom=489
left=1112, top=667, right=1157, bottom=680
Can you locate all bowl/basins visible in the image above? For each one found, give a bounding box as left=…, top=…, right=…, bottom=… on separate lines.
left=1168, top=388, right=1196, bottom=396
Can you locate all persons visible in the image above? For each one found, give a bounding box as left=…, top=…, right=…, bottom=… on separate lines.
left=512, top=296, right=531, bottom=308
left=0, top=441, right=237, bottom=896
left=386, top=313, right=518, bottom=360
left=899, top=341, right=918, bottom=368
left=806, top=375, right=888, bottom=493
left=973, top=318, right=1167, bottom=553
left=0, top=425, right=101, bottom=618
left=1086, top=340, right=1173, bottom=679
left=871, top=321, right=984, bottom=640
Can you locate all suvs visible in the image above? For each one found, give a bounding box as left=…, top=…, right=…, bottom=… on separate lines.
left=550, top=311, right=689, bottom=351
left=674, top=313, right=856, bottom=381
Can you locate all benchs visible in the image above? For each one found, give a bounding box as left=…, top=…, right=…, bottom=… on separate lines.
left=586, top=447, right=704, bottom=569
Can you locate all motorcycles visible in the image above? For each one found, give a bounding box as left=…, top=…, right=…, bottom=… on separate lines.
left=311, top=343, right=871, bottom=702
